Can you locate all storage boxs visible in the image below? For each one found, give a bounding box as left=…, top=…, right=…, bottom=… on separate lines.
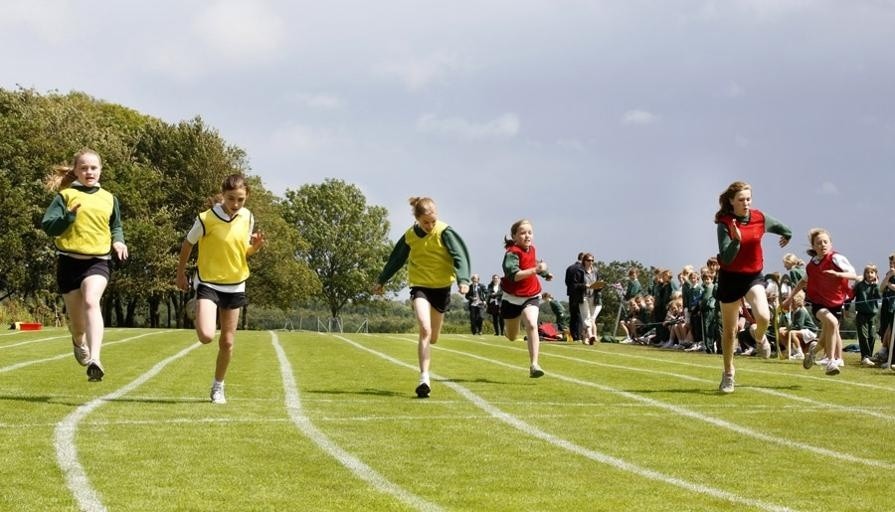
left=21, top=323, right=42, bottom=330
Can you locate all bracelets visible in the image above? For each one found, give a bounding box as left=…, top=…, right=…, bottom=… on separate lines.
left=532, top=268, right=536, bottom=275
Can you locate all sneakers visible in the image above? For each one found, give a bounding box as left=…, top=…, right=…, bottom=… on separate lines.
left=814, top=357, right=830, bottom=366
left=803, top=340, right=819, bottom=370
left=415, top=378, right=431, bottom=399
left=71, top=334, right=92, bottom=367
left=185, top=298, right=197, bottom=321
left=619, top=335, right=805, bottom=361
left=749, top=323, right=772, bottom=359
left=209, top=381, right=225, bottom=405
left=529, top=362, right=544, bottom=378
left=834, top=358, right=844, bottom=367
left=860, top=354, right=879, bottom=367
left=824, top=360, right=840, bottom=375
left=718, top=365, right=736, bottom=393
left=87, top=357, right=104, bottom=382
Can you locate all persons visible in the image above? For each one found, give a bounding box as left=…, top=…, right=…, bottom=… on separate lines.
left=565, top=252, right=584, bottom=341
left=174, top=172, right=265, bottom=406
left=465, top=275, right=487, bottom=335
left=501, top=219, right=553, bottom=378
left=714, top=181, right=792, bottom=394
left=619, top=228, right=895, bottom=377
left=40, top=148, right=129, bottom=382
left=486, top=275, right=505, bottom=336
left=572, top=253, right=603, bottom=345
left=372, top=197, right=471, bottom=398
left=541, top=292, right=567, bottom=340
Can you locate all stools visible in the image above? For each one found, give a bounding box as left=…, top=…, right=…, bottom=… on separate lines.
left=802, top=338, right=827, bottom=360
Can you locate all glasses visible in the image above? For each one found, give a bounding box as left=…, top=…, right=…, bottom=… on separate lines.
left=585, top=259, right=595, bottom=262
